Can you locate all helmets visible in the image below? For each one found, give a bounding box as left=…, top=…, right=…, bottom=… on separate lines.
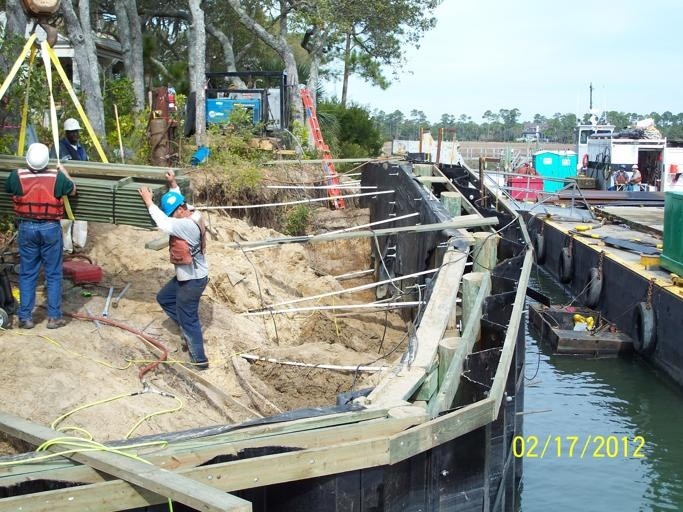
left=63, top=118, right=83, bottom=134
left=26, top=142, right=49, bottom=174
left=160, top=191, right=185, bottom=216
left=620, top=163, right=638, bottom=168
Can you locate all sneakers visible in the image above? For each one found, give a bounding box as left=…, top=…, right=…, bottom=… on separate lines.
left=47, top=320, right=65, bottom=328
left=190, top=363, right=207, bottom=371
left=18, top=320, right=33, bottom=328
left=181, top=336, right=188, bottom=352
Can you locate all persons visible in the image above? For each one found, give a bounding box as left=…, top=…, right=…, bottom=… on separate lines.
left=136, top=168, right=218, bottom=370
left=165, top=83, right=176, bottom=95
left=3, top=141, right=78, bottom=331
left=625, top=164, right=642, bottom=192
left=166, top=89, right=175, bottom=108
left=220, top=76, right=230, bottom=90
left=48, top=118, right=88, bottom=161
left=614, top=163, right=630, bottom=191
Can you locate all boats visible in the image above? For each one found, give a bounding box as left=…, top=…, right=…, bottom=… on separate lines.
left=463, top=119, right=683, bottom=387
